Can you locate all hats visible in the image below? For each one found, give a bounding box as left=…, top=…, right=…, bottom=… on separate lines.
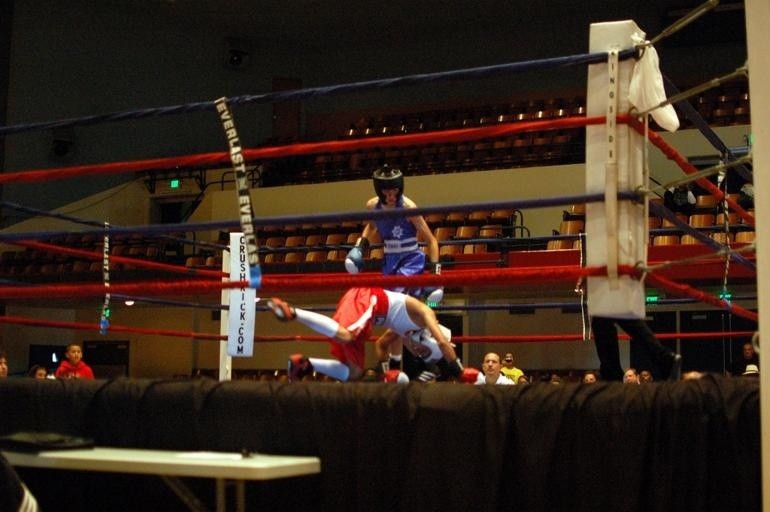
left=742, top=364, right=760, bottom=376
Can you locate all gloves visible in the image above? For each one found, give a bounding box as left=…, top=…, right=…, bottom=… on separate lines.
left=345, top=237, right=369, bottom=274
left=381, top=369, right=409, bottom=383
left=424, top=263, right=443, bottom=304
left=448, top=358, right=486, bottom=385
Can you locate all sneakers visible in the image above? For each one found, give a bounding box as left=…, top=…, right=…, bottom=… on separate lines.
left=288, top=353, right=314, bottom=384
left=266, top=296, right=296, bottom=322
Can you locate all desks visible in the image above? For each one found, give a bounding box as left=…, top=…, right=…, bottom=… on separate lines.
left=1, top=446, right=321, bottom=511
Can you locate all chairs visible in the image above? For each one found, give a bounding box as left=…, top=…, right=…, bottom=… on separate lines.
left=258, top=92, right=750, bottom=189
left=1, top=226, right=190, bottom=281
left=192, top=202, right=525, bottom=267
left=545, top=190, right=733, bottom=249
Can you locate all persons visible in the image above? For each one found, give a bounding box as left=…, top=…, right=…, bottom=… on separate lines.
left=575, top=317, right=682, bottom=383
left=267, top=286, right=485, bottom=386
left=0, top=351, right=9, bottom=379
left=475, top=343, right=758, bottom=385
left=344, top=166, right=444, bottom=382
left=29, top=343, right=95, bottom=381
left=664, top=183, right=696, bottom=216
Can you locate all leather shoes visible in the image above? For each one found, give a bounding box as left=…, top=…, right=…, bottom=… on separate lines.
left=671, top=353, right=682, bottom=381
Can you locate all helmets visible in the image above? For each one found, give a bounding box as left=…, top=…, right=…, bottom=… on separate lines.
left=412, top=323, right=451, bottom=363
left=372, top=164, right=405, bottom=205
